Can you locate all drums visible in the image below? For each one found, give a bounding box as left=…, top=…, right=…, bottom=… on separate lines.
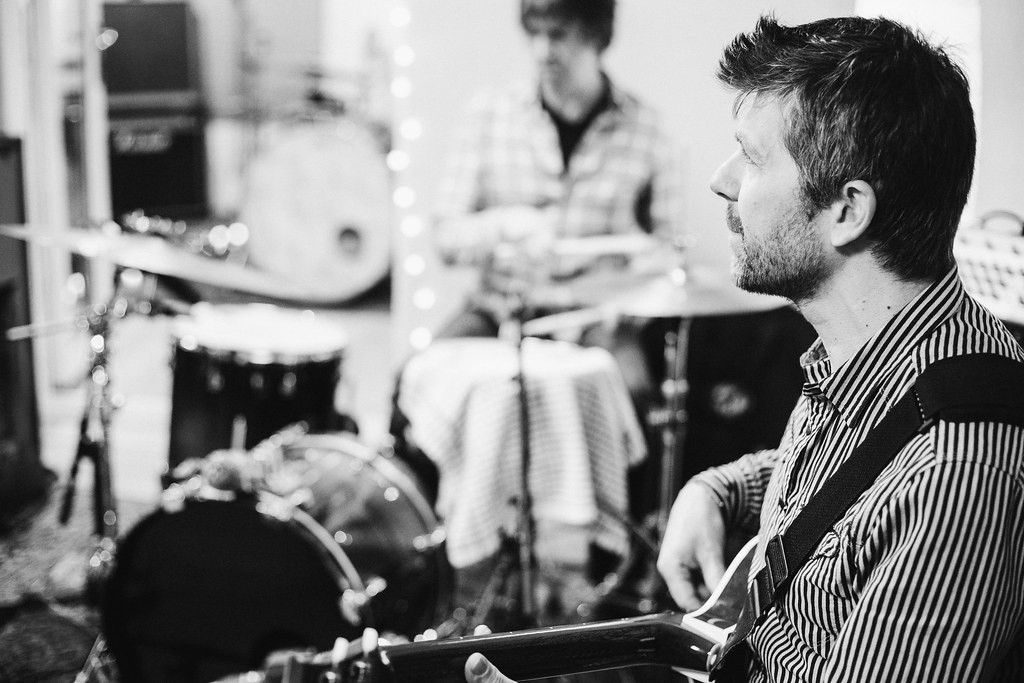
left=162, top=298, right=346, bottom=494
left=96, top=427, right=454, bottom=682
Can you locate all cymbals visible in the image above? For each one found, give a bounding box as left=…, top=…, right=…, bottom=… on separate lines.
left=604, top=260, right=792, bottom=320
left=0, top=208, right=344, bottom=311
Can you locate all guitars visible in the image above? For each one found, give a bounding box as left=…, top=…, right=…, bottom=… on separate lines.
left=264, top=533, right=761, bottom=683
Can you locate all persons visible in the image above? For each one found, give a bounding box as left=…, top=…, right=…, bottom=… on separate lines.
left=330, top=16, right=1024, bottom=683
left=389, top=1, right=679, bottom=500
